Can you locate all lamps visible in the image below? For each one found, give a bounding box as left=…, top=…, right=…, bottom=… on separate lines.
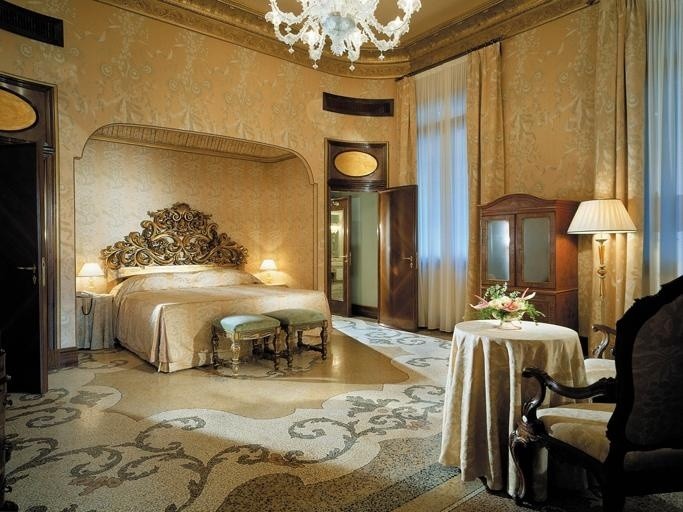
left=567, top=200, right=637, bottom=356
left=265, top=0, right=421, bottom=72
left=259, top=260, right=277, bottom=284
left=77, top=263, right=105, bottom=292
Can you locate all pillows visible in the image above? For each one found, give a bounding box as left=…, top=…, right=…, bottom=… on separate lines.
left=122, top=270, right=192, bottom=293
left=194, top=269, right=264, bottom=289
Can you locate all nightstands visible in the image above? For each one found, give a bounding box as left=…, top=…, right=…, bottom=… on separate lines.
left=267, top=284, right=288, bottom=287
left=76, top=294, right=114, bottom=350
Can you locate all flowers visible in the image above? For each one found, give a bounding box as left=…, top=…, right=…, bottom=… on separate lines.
left=469, top=282, right=545, bottom=324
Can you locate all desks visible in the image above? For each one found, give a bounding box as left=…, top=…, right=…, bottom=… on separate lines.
left=438, top=319, right=588, bottom=491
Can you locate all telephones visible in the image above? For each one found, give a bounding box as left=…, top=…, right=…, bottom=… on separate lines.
left=75, top=291, right=93, bottom=298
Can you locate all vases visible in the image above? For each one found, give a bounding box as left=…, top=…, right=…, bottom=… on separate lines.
left=492, top=310, right=524, bottom=330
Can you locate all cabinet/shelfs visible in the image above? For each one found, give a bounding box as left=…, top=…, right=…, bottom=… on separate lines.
left=475, top=193, right=580, bottom=328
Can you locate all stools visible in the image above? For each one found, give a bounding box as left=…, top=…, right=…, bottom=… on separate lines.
left=211, top=316, right=281, bottom=373
left=263, top=308, right=328, bottom=370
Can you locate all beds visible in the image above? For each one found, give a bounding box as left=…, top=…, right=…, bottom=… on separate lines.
left=101, top=202, right=332, bottom=372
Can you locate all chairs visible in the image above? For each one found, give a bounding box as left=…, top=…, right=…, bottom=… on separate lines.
left=510, top=275, right=683, bottom=512
